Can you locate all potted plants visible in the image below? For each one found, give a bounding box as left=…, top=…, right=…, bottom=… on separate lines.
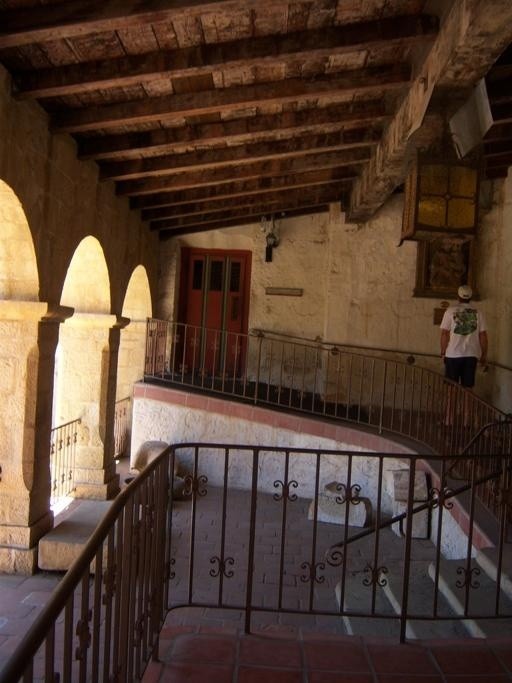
left=411, top=241, right=480, bottom=303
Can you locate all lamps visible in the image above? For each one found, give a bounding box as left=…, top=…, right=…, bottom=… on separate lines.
left=129, top=440, right=179, bottom=482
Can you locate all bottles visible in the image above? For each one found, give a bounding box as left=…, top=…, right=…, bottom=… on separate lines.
left=458, top=286, right=472, bottom=299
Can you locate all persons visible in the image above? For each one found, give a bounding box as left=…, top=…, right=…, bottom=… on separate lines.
left=440, top=284, right=488, bottom=428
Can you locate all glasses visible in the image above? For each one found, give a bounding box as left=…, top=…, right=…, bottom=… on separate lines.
left=264, top=232, right=277, bottom=262
